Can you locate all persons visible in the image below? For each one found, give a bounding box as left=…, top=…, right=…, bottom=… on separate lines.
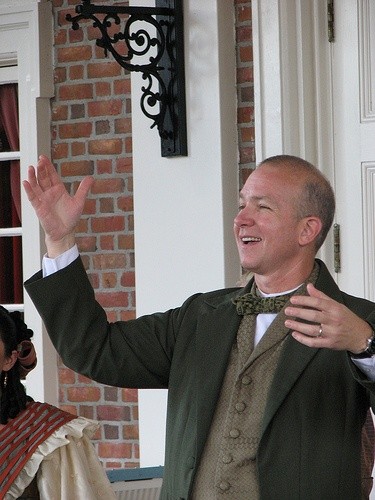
left=21, top=154, right=375, bottom=500
left=0, top=305, right=114, bottom=499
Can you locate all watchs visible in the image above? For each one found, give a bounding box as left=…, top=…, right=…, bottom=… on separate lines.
left=348, top=326, right=375, bottom=359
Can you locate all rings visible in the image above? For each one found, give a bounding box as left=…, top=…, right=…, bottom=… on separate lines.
left=319, top=323, right=324, bottom=338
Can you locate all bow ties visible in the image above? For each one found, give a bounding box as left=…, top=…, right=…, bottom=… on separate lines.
left=232, top=292, right=292, bottom=316
